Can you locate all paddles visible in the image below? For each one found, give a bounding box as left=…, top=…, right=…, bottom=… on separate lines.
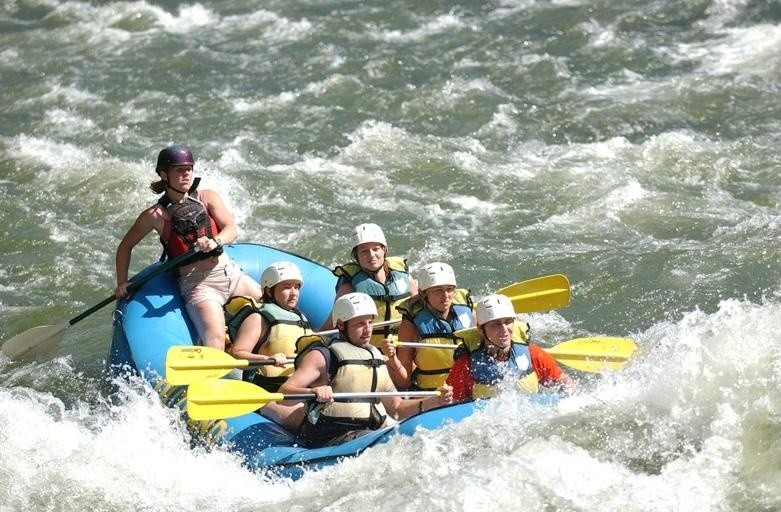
left=187, top=380, right=441, bottom=422
left=165, top=346, right=296, bottom=385
left=315, top=274, right=571, bottom=335
left=0, top=248, right=199, bottom=361
left=391, top=336, right=636, bottom=372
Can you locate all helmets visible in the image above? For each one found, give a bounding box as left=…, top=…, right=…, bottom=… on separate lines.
left=332, top=291, right=378, bottom=326
left=260, top=260, right=304, bottom=294
left=349, top=222, right=388, bottom=250
left=157, top=143, right=195, bottom=169
left=418, top=262, right=456, bottom=290
left=475, top=293, right=517, bottom=329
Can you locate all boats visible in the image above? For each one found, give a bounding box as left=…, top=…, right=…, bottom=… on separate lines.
left=106, top=242, right=570, bottom=508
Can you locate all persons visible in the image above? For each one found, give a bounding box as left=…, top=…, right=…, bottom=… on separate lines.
left=115, top=143, right=262, bottom=351
